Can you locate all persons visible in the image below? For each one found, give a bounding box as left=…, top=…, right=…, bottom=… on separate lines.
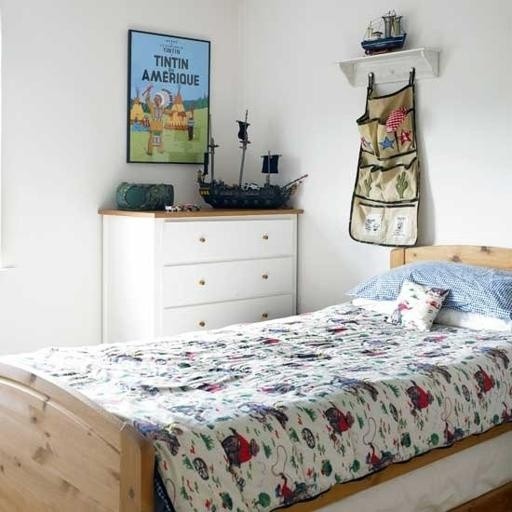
left=145, top=85, right=178, bottom=157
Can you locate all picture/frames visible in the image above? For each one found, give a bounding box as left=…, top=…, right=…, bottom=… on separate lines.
left=126, top=28, right=211, bottom=166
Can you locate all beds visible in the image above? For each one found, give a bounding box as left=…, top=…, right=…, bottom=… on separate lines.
left=0, top=243, right=511, bottom=511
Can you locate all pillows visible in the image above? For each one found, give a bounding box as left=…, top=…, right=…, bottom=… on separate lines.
left=350, top=259, right=512, bottom=334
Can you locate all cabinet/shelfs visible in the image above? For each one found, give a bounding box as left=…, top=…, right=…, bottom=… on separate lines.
left=100, top=215, right=306, bottom=340
